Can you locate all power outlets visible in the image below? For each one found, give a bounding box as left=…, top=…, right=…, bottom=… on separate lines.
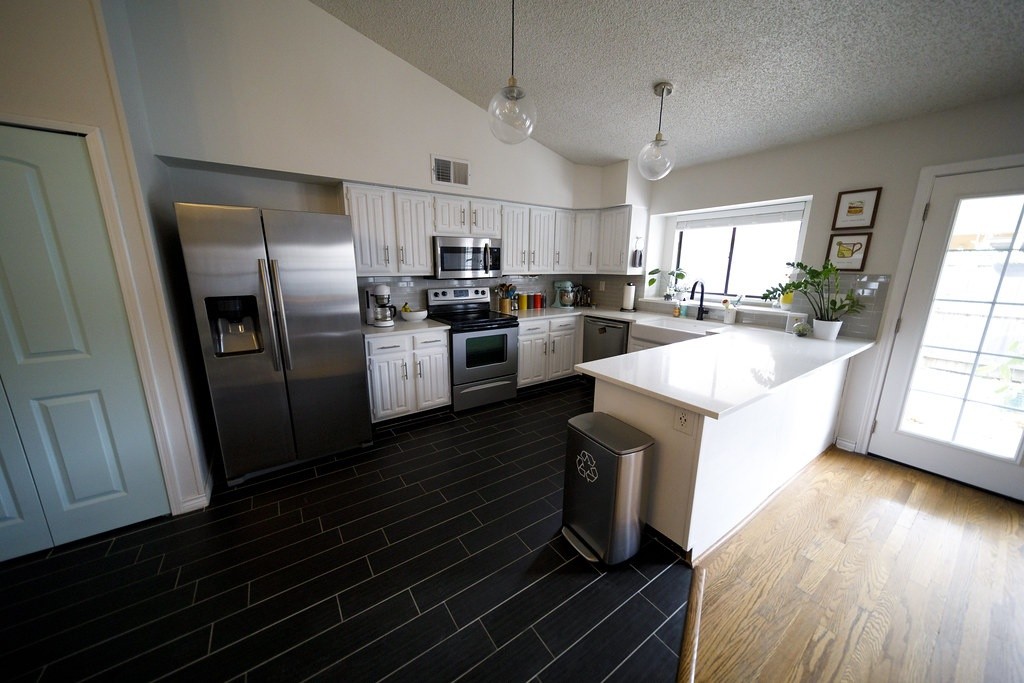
left=673, top=409, right=697, bottom=435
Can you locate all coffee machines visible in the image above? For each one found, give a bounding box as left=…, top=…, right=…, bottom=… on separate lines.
left=365, top=284, right=397, bottom=327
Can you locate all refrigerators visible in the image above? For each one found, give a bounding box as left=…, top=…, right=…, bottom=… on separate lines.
left=173, top=201, right=374, bottom=489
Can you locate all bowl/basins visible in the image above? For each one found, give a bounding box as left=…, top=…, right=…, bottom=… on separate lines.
left=400, top=309, right=427, bottom=322
left=560, top=292, right=574, bottom=306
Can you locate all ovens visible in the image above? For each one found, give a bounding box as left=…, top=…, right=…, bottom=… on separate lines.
left=449, top=325, right=517, bottom=413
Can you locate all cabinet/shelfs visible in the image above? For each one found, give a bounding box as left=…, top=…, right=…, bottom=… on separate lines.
left=361, top=330, right=450, bottom=423
left=338, top=182, right=646, bottom=280
left=516, top=316, right=583, bottom=388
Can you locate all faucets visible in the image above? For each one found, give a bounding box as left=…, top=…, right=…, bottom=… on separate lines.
left=690, top=279, right=709, bottom=320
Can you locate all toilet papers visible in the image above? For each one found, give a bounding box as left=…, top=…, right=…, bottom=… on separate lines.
left=622, top=285, right=636, bottom=309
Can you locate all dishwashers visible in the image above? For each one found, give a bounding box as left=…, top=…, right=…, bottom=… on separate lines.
left=582, top=316, right=628, bottom=364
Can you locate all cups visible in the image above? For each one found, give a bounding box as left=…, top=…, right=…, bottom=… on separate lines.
left=542, top=296, right=546, bottom=308
left=724, top=309, right=736, bottom=324
left=527, top=294, right=534, bottom=309
left=500, top=299, right=511, bottom=313
left=533, top=293, right=542, bottom=308
left=519, top=294, right=527, bottom=309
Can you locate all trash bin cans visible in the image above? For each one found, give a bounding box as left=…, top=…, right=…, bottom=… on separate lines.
left=560, top=412, right=654, bottom=565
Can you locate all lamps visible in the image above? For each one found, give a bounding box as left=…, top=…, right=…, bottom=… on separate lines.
left=487, top=1, right=536, bottom=145
left=637, top=83, right=675, bottom=181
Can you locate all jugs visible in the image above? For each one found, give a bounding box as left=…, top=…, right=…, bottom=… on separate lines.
left=374, top=305, right=396, bottom=321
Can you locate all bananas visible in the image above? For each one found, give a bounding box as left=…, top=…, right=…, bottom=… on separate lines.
left=402, top=302, right=414, bottom=312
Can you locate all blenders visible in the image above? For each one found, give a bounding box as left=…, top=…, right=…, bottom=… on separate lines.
left=551, top=280, right=575, bottom=309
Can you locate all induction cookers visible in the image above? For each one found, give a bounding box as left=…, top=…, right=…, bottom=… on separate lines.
left=426, top=287, right=519, bottom=333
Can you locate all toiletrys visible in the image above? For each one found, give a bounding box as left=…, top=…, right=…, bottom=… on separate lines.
left=679, top=297, right=688, bottom=317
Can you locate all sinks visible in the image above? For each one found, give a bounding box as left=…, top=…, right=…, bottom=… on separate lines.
left=631, top=318, right=725, bottom=344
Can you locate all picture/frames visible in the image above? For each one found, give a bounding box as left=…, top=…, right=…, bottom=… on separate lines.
left=829, top=187, right=882, bottom=230
left=823, top=232, right=873, bottom=272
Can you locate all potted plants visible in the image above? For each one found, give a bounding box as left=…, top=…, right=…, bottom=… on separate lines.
left=646, top=267, right=686, bottom=296
left=761, top=257, right=868, bottom=340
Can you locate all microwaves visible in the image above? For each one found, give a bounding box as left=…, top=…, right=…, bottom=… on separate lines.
left=433, top=235, right=502, bottom=280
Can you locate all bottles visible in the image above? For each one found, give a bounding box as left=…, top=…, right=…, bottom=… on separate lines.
left=680, top=298, right=687, bottom=317
left=576, top=283, right=591, bottom=307
left=673, top=300, right=680, bottom=317
